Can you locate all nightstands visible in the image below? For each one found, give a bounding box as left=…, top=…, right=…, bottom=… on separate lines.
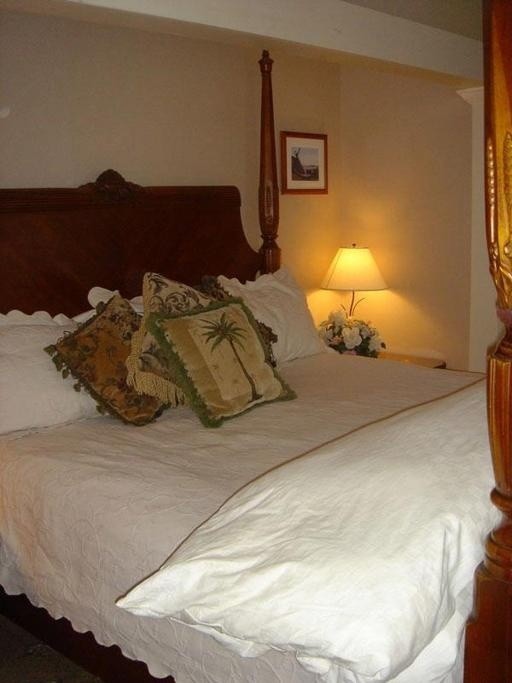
left=376, top=345, right=447, bottom=371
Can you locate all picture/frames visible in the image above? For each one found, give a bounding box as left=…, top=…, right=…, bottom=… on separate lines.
left=278, top=126, right=329, bottom=195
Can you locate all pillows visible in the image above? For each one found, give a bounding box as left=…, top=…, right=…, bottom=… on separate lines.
left=129, top=268, right=278, bottom=407
left=0, top=300, right=102, bottom=438
left=146, top=292, right=301, bottom=428
left=219, top=266, right=330, bottom=364
left=42, top=289, right=160, bottom=428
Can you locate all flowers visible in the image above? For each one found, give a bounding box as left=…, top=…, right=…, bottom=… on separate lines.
left=316, top=312, right=386, bottom=357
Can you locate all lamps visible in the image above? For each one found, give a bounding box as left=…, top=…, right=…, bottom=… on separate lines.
left=321, top=240, right=390, bottom=319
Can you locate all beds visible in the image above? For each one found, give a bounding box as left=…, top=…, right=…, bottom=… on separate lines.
left=0, top=167, right=511, bottom=678
